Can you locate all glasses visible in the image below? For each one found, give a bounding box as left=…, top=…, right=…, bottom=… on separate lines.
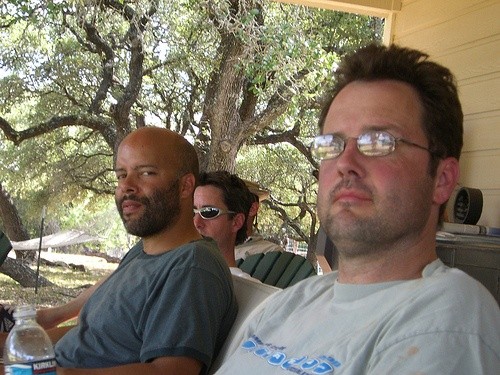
left=193, top=207, right=237, bottom=220
left=310, top=129, right=431, bottom=160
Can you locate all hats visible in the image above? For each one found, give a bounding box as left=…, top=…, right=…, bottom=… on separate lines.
left=243, top=180, right=269, bottom=202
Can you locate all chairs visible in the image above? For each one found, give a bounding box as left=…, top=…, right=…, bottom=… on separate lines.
left=209, top=251, right=316, bottom=375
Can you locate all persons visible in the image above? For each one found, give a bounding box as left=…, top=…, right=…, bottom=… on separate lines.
left=210, top=42, right=500, bottom=375
left=192, top=169, right=284, bottom=284
left=0, top=127, right=239, bottom=375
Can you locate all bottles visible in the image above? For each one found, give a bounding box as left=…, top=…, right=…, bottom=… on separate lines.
left=4, top=304, right=56, bottom=375
